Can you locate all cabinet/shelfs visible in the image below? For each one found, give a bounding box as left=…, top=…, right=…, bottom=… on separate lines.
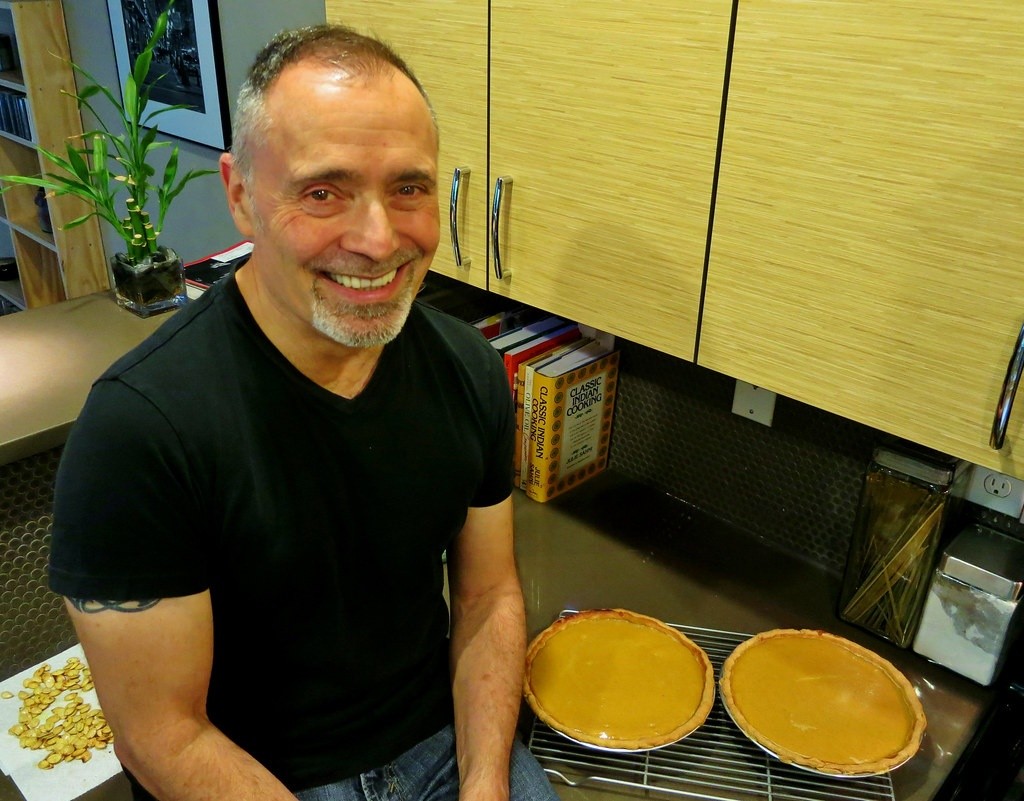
left=0, top=0, right=110, bottom=316
left=319, top=0, right=1024, bottom=481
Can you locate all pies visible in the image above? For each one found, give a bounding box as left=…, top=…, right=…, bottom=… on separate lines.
left=521, top=608, right=715, bottom=750
left=719, top=628, right=927, bottom=773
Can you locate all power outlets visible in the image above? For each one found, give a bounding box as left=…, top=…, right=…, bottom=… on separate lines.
left=965, top=464, right=1024, bottom=518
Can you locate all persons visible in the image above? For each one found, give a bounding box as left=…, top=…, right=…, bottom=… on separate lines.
left=47, top=25, right=559, bottom=801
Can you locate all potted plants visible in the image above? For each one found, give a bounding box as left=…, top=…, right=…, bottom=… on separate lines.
left=0, top=0, right=222, bottom=318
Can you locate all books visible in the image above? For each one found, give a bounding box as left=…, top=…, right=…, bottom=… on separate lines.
left=0, top=91, right=35, bottom=142
left=470, top=312, right=620, bottom=502
left=184, top=240, right=255, bottom=300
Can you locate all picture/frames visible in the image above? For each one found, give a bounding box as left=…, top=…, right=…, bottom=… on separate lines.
left=106, top=0, right=233, bottom=152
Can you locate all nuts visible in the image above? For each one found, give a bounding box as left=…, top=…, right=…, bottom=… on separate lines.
left=2, top=656, right=115, bottom=768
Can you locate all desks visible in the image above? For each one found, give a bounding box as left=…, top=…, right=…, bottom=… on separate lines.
left=0, top=260, right=993, bottom=801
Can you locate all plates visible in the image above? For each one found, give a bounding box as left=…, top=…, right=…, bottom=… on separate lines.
left=716, top=651, right=926, bottom=778
left=525, top=617, right=714, bottom=753
